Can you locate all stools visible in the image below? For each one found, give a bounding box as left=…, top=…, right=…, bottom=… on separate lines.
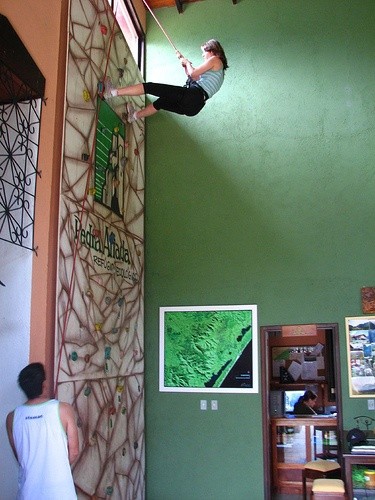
left=302, top=459, right=341, bottom=500
left=311, top=479, right=347, bottom=500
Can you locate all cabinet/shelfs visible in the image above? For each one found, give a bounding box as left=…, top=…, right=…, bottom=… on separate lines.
left=271, top=417, right=337, bottom=493
left=342, top=449, right=375, bottom=500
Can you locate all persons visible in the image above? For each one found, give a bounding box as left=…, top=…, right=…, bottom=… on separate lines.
left=293, top=390, right=319, bottom=415
left=102, top=40, right=228, bottom=123
left=7, top=363, right=80, bottom=500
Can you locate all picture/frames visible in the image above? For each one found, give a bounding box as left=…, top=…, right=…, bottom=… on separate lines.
left=345, top=316, right=375, bottom=398
left=158, top=304, right=259, bottom=394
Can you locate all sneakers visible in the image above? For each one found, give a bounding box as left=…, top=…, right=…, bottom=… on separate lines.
left=103, top=75, right=113, bottom=100
left=127, top=101, right=135, bottom=123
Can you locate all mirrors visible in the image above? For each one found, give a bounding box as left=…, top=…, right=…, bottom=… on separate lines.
left=257, top=322, right=343, bottom=500
